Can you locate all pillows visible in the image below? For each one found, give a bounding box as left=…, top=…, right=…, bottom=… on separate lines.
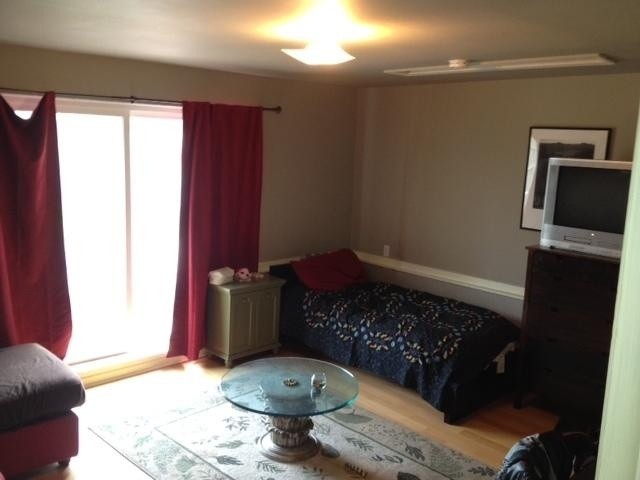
left=292, top=247, right=374, bottom=293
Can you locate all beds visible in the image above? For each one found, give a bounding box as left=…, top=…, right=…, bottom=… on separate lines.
left=269, top=263, right=520, bottom=428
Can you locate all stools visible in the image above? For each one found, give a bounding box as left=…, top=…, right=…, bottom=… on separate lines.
left=2, top=341, right=87, bottom=480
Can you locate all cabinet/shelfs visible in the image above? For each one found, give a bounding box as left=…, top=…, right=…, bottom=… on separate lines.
left=512, top=243, right=620, bottom=430
left=204, top=273, right=288, bottom=369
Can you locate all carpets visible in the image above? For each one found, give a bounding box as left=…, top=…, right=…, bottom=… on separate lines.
left=90, top=368, right=501, bottom=479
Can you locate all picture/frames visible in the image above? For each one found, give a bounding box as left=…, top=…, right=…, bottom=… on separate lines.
left=521, top=126, right=613, bottom=233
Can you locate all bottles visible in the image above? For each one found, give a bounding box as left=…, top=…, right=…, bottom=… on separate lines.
left=311, top=372, right=327, bottom=389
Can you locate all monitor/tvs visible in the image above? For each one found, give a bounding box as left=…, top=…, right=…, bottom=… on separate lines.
left=540, top=157, right=632, bottom=258
